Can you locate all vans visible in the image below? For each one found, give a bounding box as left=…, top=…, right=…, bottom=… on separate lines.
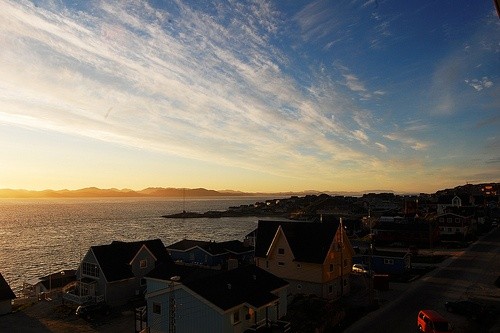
left=418, top=310, right=450, bottom=333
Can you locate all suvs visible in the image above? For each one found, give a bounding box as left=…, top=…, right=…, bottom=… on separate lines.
left=352, top=264, right=375, bottom=278
